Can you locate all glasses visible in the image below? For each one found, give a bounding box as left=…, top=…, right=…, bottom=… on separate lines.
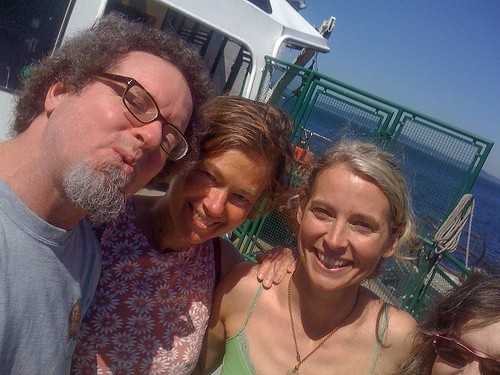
left=432, top=331, right=500, bottom=375
left=89, top=70, right=191, bottom=161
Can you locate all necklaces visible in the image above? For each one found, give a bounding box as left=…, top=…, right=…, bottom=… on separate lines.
left=287, top=269, right=360, bottom=375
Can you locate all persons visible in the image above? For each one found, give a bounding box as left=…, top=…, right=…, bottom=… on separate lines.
left=199, top=140, right=424, bottom=375
left=0, top=13, right=214, bottom=375
left=397, top=269, right=500, bottom=375
left=68, top=93, right=298, bottom=375
